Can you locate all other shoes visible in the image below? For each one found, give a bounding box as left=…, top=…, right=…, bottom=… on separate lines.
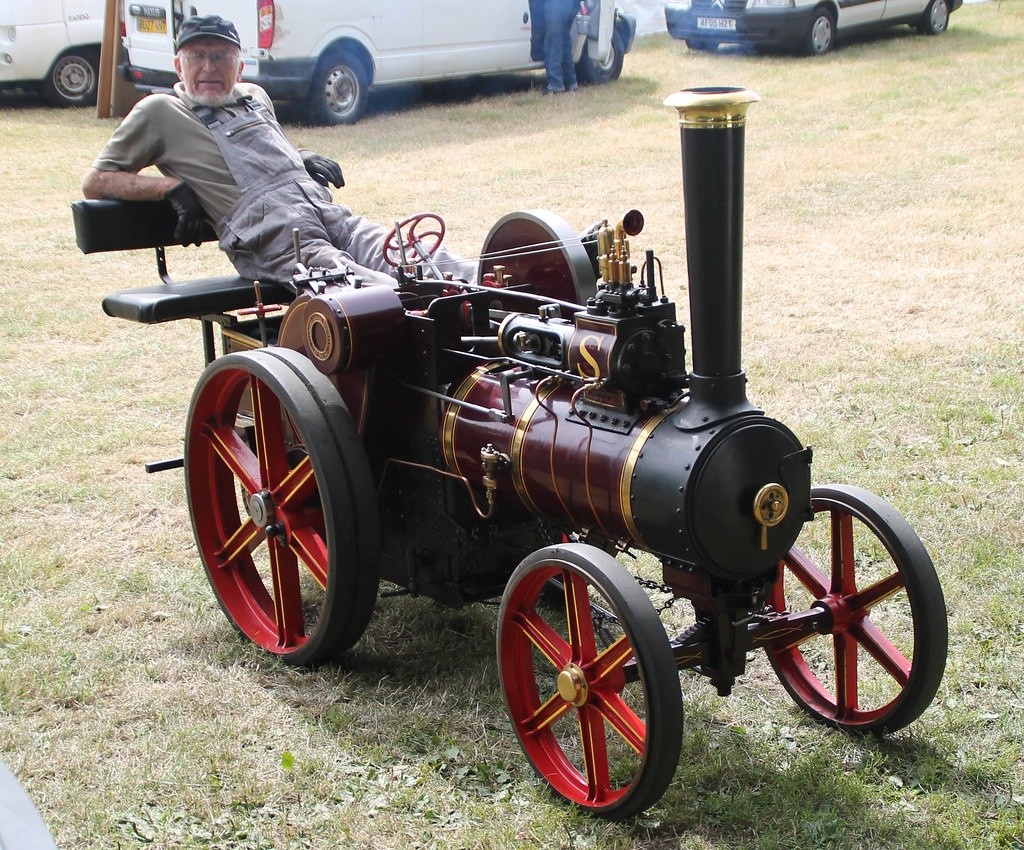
left=541, top=88, right=565, bottom=95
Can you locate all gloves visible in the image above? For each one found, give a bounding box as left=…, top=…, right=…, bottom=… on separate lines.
left=163, top=182, right=207, bottom=247
left=300, top=150, right=345, bottom=189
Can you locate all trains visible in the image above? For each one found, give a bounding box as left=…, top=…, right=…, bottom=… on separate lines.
left=70, top=86, right=949, bottom=818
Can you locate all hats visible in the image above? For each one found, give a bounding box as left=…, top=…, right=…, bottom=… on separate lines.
left=176, top=14, right=241, bottom=52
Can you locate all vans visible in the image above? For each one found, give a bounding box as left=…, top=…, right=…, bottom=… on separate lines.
left=665, top=1, right=964, bottom=56
left=121, top=1, right=636, bottom=126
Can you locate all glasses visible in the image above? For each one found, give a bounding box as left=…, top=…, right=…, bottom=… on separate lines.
left=180, top=51, right=242, bottom=66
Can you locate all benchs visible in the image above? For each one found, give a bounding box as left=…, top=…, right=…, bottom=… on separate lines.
left=72, top=198, right=290, bottom=323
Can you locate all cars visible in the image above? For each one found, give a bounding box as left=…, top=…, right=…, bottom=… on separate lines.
left=1, top=0, right=104, bottom=106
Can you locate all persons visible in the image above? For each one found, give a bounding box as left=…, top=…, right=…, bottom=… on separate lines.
left=542, top=0, right=577, bottom=95
left=81, top=14, right=479, bottom=295
